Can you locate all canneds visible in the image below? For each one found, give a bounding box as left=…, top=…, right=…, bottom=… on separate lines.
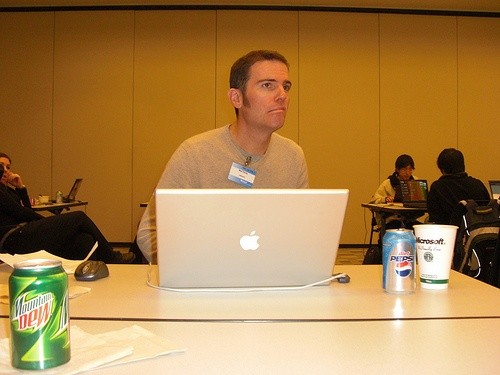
left=8, top=259, right=70, bottom=370
left=382, top=228, right=417, bottom=295
left=56, top=191, right=63, bottom=204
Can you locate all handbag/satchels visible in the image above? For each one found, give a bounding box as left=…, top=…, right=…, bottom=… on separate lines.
left=363, top=246, right=383, bottom=265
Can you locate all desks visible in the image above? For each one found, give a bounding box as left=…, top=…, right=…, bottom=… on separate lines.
left=21, top=201, right=88, bottom=219
left=0, top=265, right=500, bottom=375
left=367, top=203, right=429, bottom=241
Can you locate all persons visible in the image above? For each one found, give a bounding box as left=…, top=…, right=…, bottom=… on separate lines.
left=131, top=48, right=310, bottom=266
left=0, top=152, right=137, bottom=266
left=424, top=148, right=491, bottom=265
left=371, top=154, right=425, bottom=254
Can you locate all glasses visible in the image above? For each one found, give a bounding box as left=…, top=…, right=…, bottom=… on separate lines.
left=400, top=168, right=415, bottom=172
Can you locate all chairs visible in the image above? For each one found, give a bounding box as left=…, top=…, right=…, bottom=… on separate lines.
left=369, top=195, right=500, bottom=272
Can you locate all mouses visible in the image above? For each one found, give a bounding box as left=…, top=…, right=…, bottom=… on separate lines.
left=74, top=261, right=109, bottom=281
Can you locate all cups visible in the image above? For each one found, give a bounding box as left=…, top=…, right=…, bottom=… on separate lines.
left=39, top=196, right=49, bottom=203
left=413, top=224, right=459, bottom=290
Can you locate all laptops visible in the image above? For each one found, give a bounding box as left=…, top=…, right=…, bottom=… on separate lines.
left=488, top=180, right=500, bottom=200
left=49, top=179, right=82, bottom=203
left=155, top=188, right=350, bottom=290
left=391, top=179, right=429, bottom=208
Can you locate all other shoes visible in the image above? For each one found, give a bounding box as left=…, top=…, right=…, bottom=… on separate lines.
left=121, top=252, right=136, bottom=263
